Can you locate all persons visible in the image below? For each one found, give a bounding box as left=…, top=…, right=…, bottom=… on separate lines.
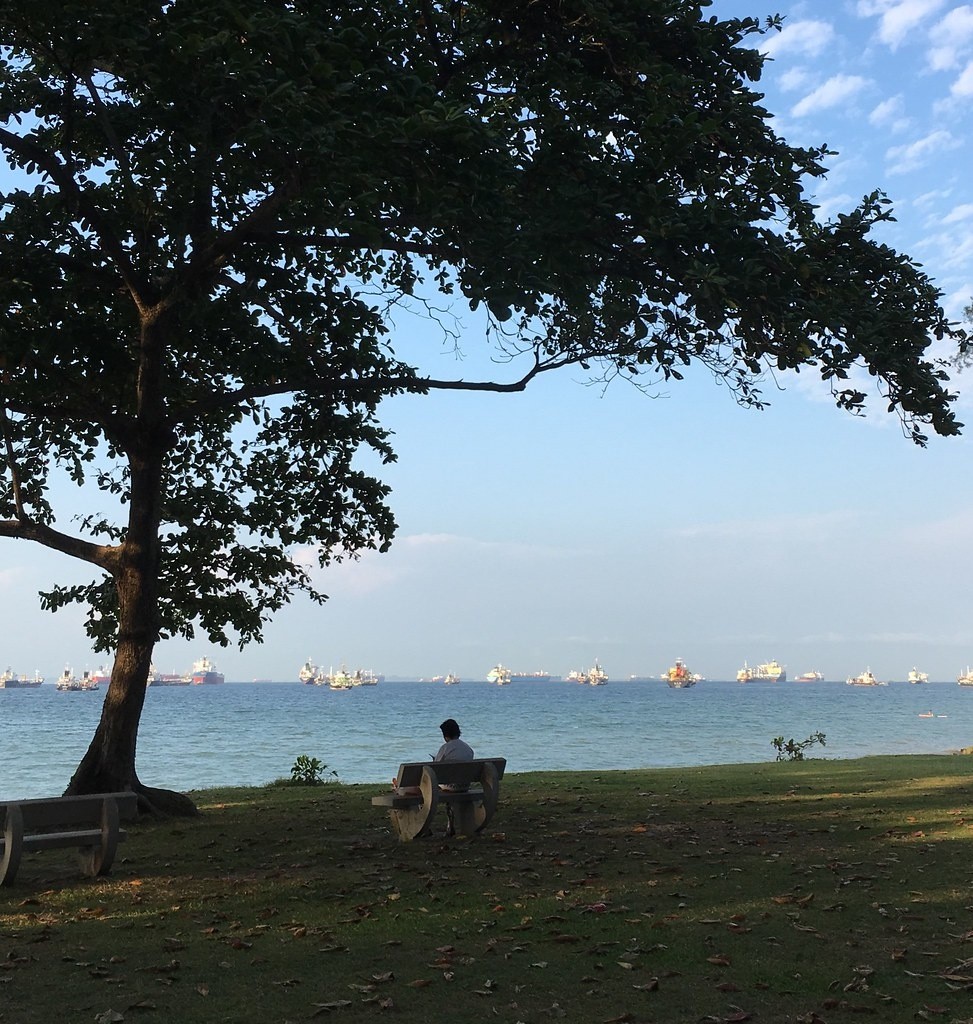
left=928, top=710, right=932, bottom=715
left=392, top=719, right=474, bottom=795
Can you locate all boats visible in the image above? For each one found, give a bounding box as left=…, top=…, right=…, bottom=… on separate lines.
left=577, top=666, right=589, bottom=684
left=737, top=658, right=787, bottom=684
left=907, top=666, right=929, bottom=684
left=625, top=673, right=641, bottom=683
left=327, top=665, right=385, bottom=690
left=299, top=657, right=319, bottom=684
left=487, top=665, right=561, bottom=683
left=432, top=673, right=445, bottom=683
left=846, top=665, right=889, bottom=687
left=0, top=666, right=44, bottom=688
left=588, top=659, right=608, bottom=685
left=313, top=666, right=329, bottom=686
left=443, top=673, right=460, bottom=686
left=192, top=655, right=225, bottom=684
left=955, top=665, right=973, bottom=686
left=666, top=656, right=696, bottom=688
left=496, top=666, right=511, bottom=686
left=565, top=670, right=578, bottom=681
left=918, top=714, right=946, bottom=718
left=88, top=663, right=193, bottom=688
left=661, top=673, right=705, bottom=682
left=794, top=666, right=824, bottom=682
left=56, top=662, right=100, bottom=691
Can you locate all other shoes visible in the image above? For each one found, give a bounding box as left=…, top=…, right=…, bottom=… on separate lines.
left=420, top=829, right=433, bottom=838
left=443, top=827, right=455, bottom=837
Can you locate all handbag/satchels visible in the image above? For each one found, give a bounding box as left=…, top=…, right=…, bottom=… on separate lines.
left=392, top=778, right=421, bottom=796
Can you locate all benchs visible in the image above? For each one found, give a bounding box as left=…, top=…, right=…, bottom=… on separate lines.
left=0, top=792, right=139, bottom=887
left=372, top=756, right=508, bottom=840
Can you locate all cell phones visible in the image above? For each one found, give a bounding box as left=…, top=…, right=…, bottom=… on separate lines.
left=429, top=754, right=436, bottom=759
